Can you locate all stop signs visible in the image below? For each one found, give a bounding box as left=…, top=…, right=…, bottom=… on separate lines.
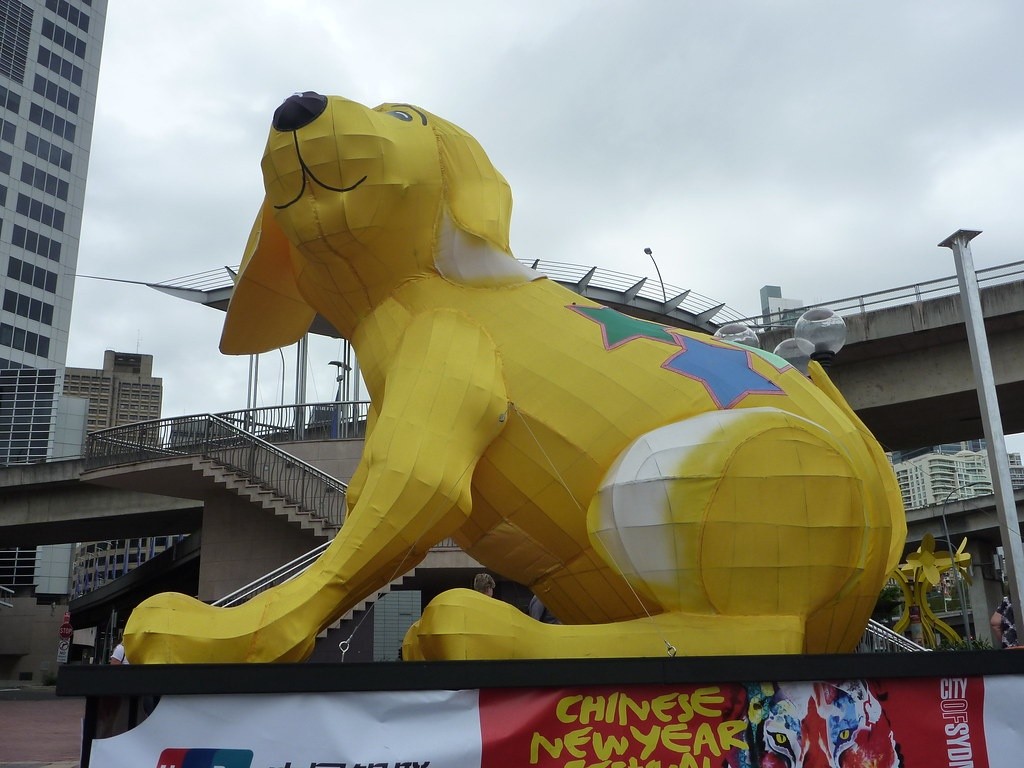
left=58, top=624, right=74, bottom=640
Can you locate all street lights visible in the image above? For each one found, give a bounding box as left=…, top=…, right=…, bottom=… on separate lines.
left=942, top=480, right=994, bottom=654
left=643, top=245, right=669, bottom=306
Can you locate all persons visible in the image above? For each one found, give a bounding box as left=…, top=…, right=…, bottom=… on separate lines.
left=990, top=595, right=1018, bottom=649
left=474, top=574, right=496, bottom=597
left=529, top=596, right=562, bottom=625
left=110, top=640, right=130, bottom=664
